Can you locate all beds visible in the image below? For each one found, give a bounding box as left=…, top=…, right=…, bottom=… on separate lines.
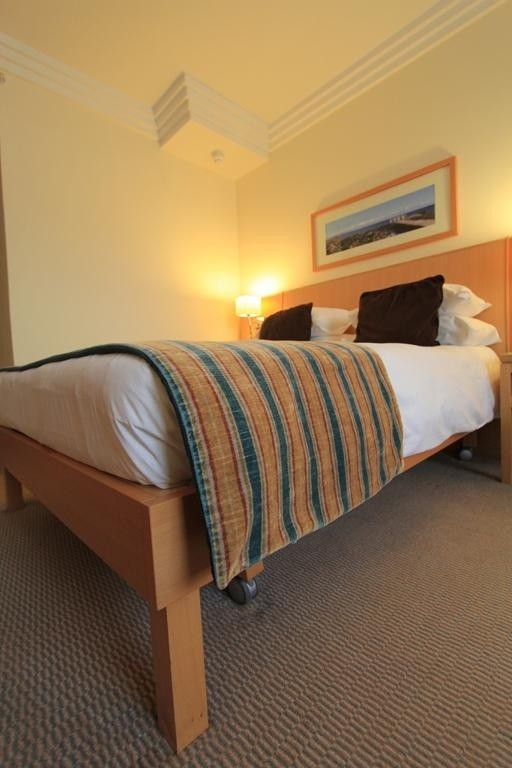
left=0, top=231, right=512, bottom=757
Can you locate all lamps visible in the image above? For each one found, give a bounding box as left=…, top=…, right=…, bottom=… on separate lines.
left=230, top=290, right=259, bottom=341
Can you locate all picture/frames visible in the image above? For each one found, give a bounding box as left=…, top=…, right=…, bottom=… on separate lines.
left=306, top=154, right=461, bottom=272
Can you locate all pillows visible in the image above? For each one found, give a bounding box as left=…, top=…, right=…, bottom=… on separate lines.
left=437, top=315, right=505, bottom=347
left=254, top=299, right=318, bottom=344
left=439, top=281, right=495, bottom=317
left=311, top=304, right=353, bottom=337
left=352, top=275, right=448, bottom=347
